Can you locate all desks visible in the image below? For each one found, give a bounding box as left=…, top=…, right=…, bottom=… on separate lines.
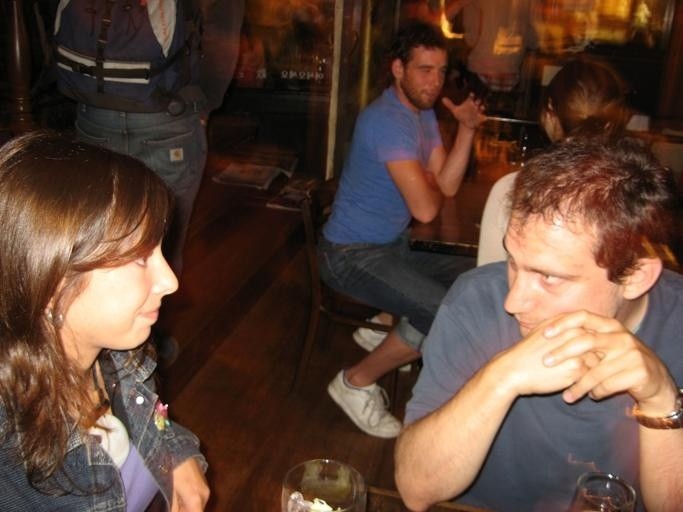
left=411, top=176, right=495, bottom=257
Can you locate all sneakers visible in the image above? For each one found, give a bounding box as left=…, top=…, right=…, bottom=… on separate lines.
left=352, top=320, right=411, bottom=373
left=327, top=369, right=403, bottom=438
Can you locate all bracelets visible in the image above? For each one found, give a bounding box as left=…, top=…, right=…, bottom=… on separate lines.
left=628, top=389, right=682, bottom=432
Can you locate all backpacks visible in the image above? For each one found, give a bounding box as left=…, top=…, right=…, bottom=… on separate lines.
left=51, top=0, right=189, bottom=112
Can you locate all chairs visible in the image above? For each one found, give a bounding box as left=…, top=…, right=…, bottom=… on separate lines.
left=295, top=177, right=421, bottom=414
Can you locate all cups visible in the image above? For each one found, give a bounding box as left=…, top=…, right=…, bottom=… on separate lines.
left=565, top=471, right=637, bottom=511
left=280, top=458, right=368, bottom=512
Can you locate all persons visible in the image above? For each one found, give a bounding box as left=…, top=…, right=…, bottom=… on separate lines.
left=393, top=121, right=682, bottom=512
left=0, top=132, right=213, bottom=511
left=473, top=53, right=637, bottom=269
left=316, top=24, right=487, bottom=443
left=24, top=0, right=246, bottom=306
